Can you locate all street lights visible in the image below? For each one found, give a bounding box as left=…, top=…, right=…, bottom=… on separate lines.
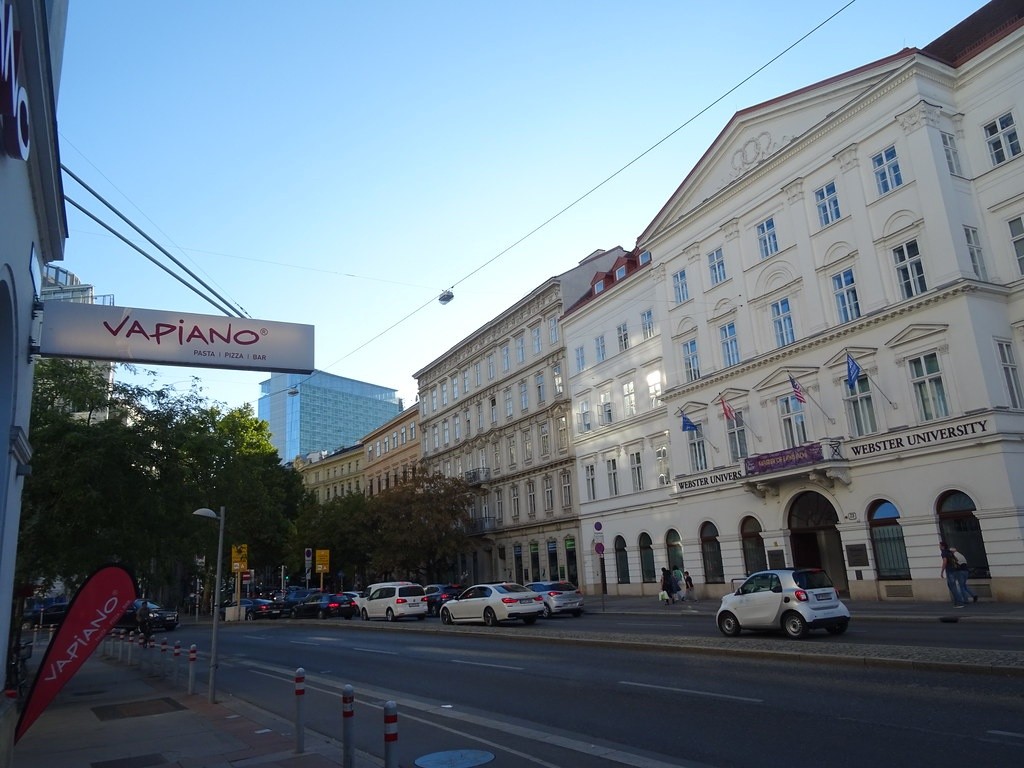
left=192, top=505, right=227, bottom=703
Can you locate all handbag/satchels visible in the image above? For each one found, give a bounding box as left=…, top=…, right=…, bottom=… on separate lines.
left=658, top=590, right=670, bottom=601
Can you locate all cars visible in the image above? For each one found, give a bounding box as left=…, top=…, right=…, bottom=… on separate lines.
left=119, top=599, right=179, bottom=630
left=716, top=569, right=853, bottom=640
left=219, top=599, right=282, bottom=621
left=424, top=585, right=459, bottom=616
left=440, top=579, right=586, bottom=627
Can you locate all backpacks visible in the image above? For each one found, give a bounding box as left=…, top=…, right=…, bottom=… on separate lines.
left=943, top=551, right=959, bottom=571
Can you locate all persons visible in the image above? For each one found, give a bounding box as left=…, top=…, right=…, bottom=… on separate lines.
left=661, top=565, right=700, bottom=606
left=939, top=541, right=978, bottom=608
left=136, top=601, right=152, bottom=635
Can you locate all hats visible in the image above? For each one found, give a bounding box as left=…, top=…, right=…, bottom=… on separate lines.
left=950, top=547, right=956, bottom=552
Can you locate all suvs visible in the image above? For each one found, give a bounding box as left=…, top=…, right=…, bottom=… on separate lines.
left=277, top=581, right=430, bottom=621
left=23, top=596, right=73, bottom=622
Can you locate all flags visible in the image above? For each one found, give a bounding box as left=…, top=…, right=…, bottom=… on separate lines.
left=680, top=410, right=698, bottom=431
left=788, top=374, right=807, bottom=403
left=847, top=353, right=861, bottom=390
left=720, top=395, right=737, bottom=420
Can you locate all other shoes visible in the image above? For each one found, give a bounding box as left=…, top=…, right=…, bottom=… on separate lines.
left=672, top=599, right=675, bottom=604
left=973, top=595, right=978, bottom=602
left=694, top=600, right=699, bottom=603
left=964, top=602, right=968, bottom=607
left=665, top=603, right=669, bottom=605
left=682, top=597, right=684, bottom=601
left=953, top=603, right=964, bottom=609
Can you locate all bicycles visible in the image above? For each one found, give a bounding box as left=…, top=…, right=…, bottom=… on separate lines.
left=142, top=618, right=160, bottom=648
left=17, top=640, right=34, bottom=687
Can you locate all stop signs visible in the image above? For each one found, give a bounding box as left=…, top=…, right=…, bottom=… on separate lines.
left=238, top=570, right=253, bottom=584
